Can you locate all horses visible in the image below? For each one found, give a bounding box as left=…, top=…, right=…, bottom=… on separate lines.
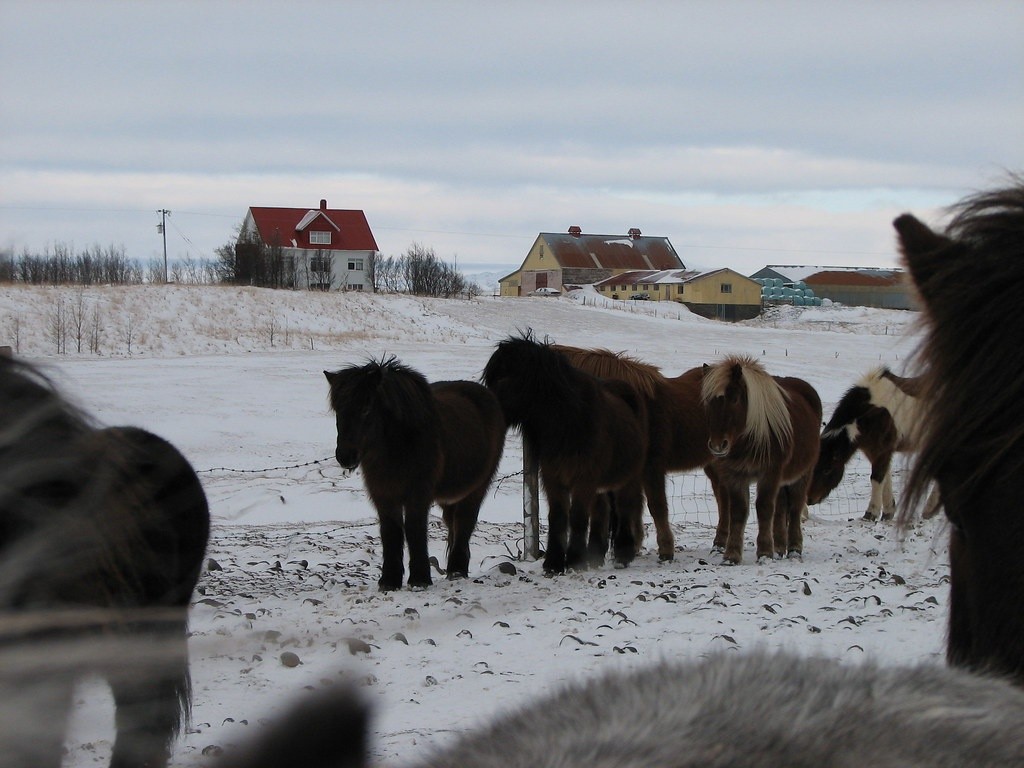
left=201, top=642, right=1024, bottom=768
left=806, top=359, right=928, bottom=522
left=484, top=338, right=651, bottom=575
left=545, top=343, right=823, bottom=566
left=0, top=352, right=211, bottom=768
left=892, top=186, right=1024, bottom=689
left=322, top=350, right=507, bottom=591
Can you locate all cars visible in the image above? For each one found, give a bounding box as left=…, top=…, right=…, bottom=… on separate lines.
left=526, top=288, right=561, bottom=298
